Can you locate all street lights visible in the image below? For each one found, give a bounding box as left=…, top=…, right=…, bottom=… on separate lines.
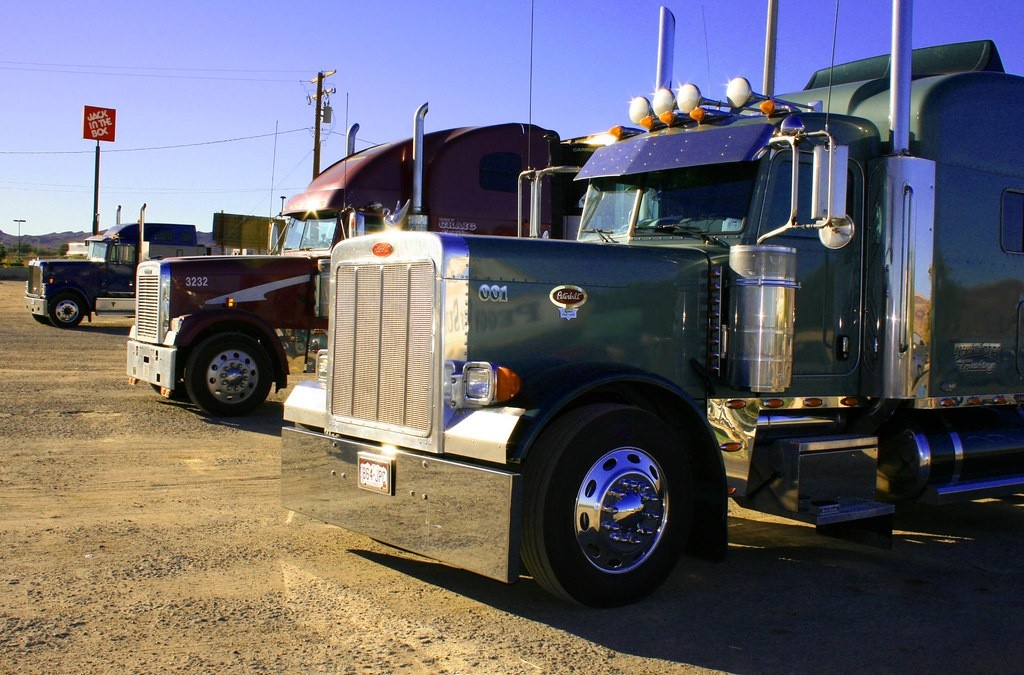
left=13, top=219, right=27, bottom=264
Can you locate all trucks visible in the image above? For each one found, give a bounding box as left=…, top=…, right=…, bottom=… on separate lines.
left=127, top=102, right=645, bottom=422
left=25, top=203, right=211, bottom=330
left=279, top=0, right=1024, bottom=610
